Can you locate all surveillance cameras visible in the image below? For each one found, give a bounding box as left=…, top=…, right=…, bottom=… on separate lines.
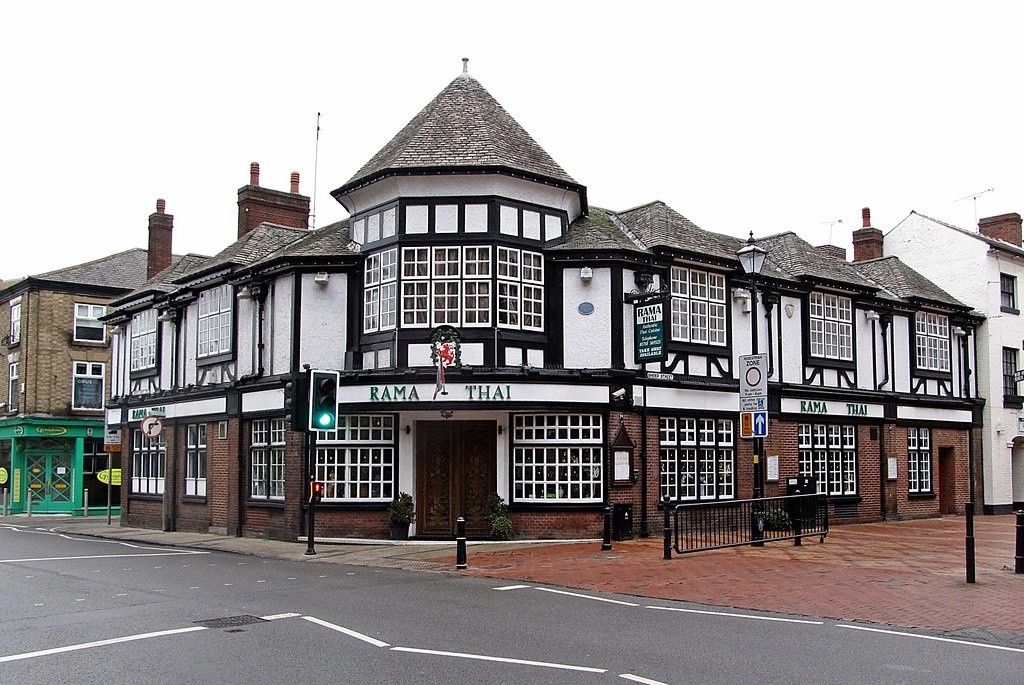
left=612, top=388, right=626, bottom=398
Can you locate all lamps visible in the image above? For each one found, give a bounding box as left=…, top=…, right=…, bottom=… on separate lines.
left=313, top=272, right=330, bottom=284
left=864, top=310, right=880, bottom=319
left=156, top=311, right=170, bottom=322
left=953, top=326, right=967, bottom=336
left=581, top=266, right=593, bottom=280
left=236, top=286, right=251, bottom=299
left=733, top=288, right=748, bottom=300
left=108, top=324, right=122, bottom=335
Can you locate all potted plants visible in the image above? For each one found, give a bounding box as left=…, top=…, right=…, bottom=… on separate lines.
left=387, top=492, right=415, bottom=540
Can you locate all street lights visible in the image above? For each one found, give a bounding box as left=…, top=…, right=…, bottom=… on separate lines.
left=735, top=230, right=770, bottom=549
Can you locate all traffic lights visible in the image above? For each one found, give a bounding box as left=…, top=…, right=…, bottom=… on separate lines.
left=283, top=378, right=295, bottom=433
left=309, top=481, right=322, bottom=506
left=308, top=370, right=340, bottom=433
